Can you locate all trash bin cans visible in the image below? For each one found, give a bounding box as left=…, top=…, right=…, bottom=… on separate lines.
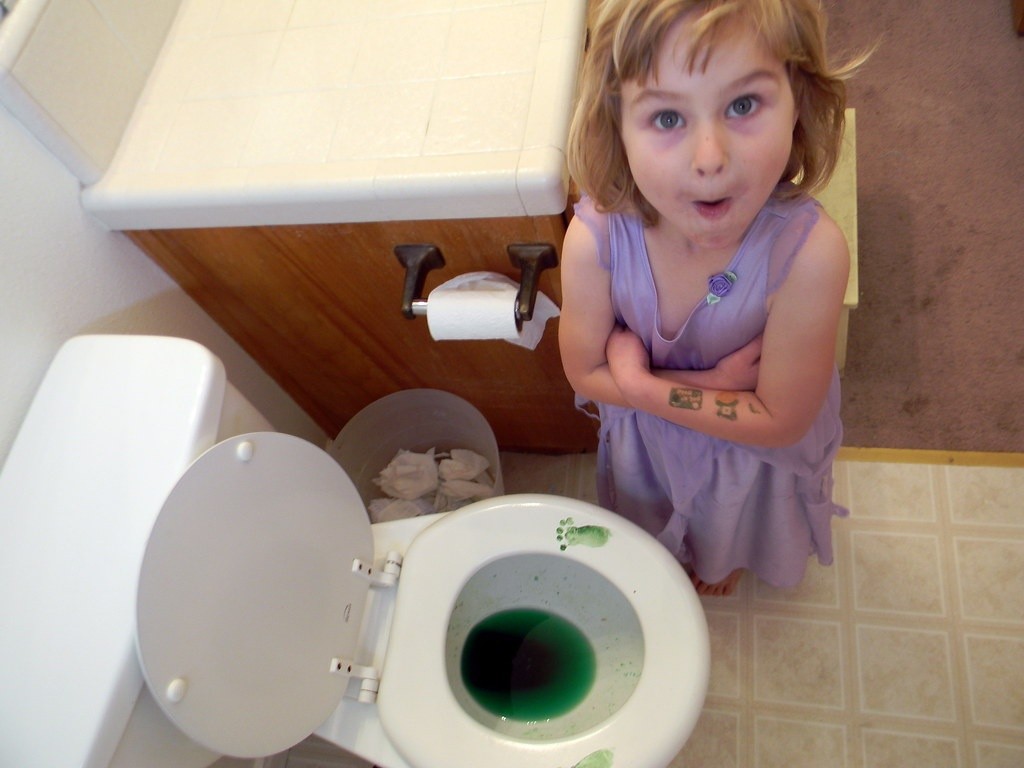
left=325, top=387, right=504, bottom=525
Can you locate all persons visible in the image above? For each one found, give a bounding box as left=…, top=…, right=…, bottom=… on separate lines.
left=557, top=0, right=851, bottom=600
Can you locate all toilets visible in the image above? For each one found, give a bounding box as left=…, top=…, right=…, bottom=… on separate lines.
left=0, top=333, right=710, bottom=767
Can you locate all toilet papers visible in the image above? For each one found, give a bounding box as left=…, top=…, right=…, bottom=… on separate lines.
left=426, top=268, right=560, bottom=353
